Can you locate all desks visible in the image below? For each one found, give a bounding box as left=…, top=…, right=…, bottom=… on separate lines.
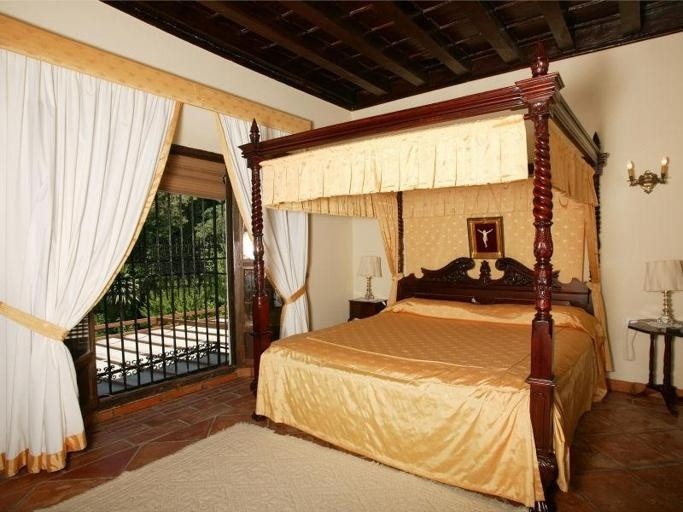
left=627, top=318, right=683, bottom=417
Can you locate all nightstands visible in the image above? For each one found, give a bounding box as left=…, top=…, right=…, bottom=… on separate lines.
left=347, top=296, right=388, bottom=323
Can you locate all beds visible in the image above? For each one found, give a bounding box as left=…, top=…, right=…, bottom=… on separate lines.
left=252, top=255, right=592, bottom=512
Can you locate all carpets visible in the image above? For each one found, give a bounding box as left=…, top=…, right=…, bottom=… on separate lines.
left=33, top=419, right=534, bottom=512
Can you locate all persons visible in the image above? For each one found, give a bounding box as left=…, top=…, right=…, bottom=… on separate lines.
left=478, top=229, right=493, bottom=247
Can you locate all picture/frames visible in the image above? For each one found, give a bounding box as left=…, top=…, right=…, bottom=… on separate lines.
left=466, top=216, right=505, bottom=260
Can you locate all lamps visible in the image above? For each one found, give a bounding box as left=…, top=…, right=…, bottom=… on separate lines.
left=642, top=258, right=683, bottom=329
left=624, top=156, right=669, bottom=193
left=358, top=256, right=383, bottom=299
